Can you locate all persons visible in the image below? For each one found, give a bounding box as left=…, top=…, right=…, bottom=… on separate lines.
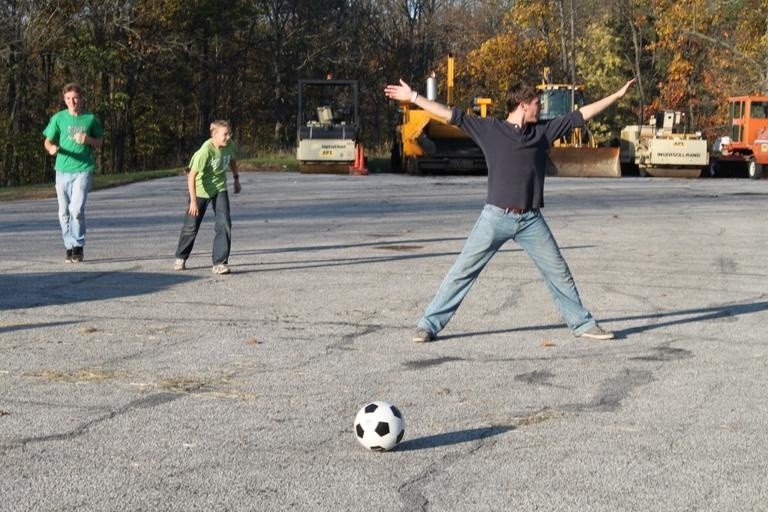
left=41, top=83, right=106, bottom=263
left=381, top=74, right=640, bottom=343
left=173, top=120, right=242, bottom=275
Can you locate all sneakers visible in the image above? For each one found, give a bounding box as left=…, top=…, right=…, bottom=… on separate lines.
left=66, top=246, right=83, bottom=263
left=581, top=325, right=614, bottom=339
left=175, top=258, right=185, bottom=269
left=414, top=328, right=431, bottom=342
left=213, top=263, right=229, bottom=273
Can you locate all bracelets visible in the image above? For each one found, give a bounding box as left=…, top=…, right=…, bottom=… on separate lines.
left=233, top=174, right=240, bottom=180
left=409, top=91, right=418, bottom=103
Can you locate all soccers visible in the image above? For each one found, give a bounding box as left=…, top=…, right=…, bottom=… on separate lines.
left=354, top=401, right=405, bottom=451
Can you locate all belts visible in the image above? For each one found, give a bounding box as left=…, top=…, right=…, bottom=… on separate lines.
left=502, top=207, right=532, bottom=213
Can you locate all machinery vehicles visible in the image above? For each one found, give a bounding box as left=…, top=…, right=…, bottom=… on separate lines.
left=391, top=52, right=495, bottom=176
left=536, top=66, right=767, bottom=178
left=296, top=72, right=364, bottom=174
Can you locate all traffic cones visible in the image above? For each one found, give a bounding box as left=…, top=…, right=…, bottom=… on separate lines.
left=349, top=143, right=369, bottom=176
